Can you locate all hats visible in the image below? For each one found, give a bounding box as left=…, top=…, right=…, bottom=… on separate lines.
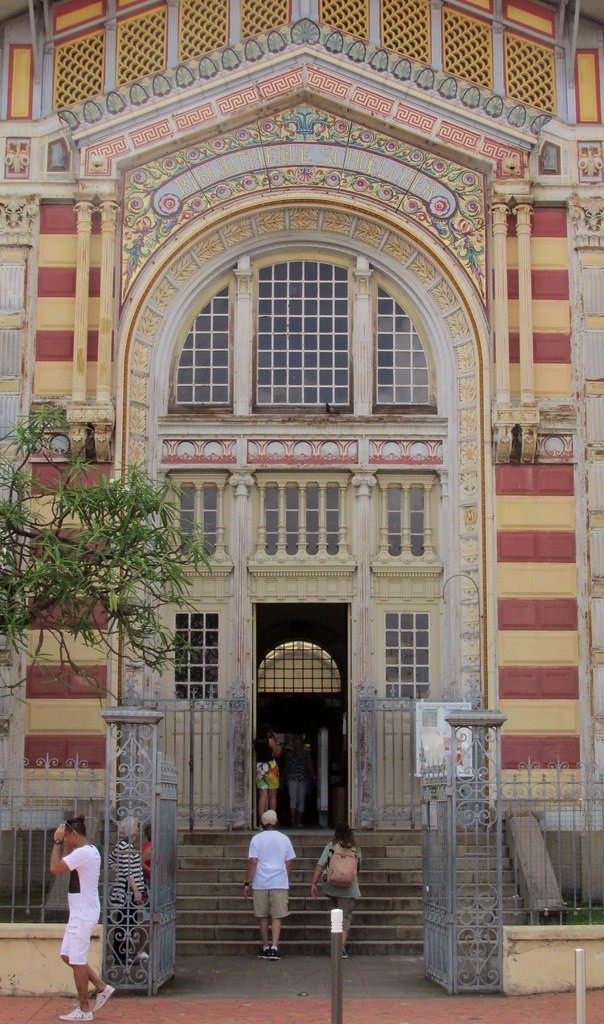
left=262, top=809, right=277, bottom=825
left=335, top=823, right=352, bottom=833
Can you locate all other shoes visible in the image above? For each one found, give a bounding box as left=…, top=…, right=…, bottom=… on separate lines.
left=135, top=951, right=153, bottom=960
left=117, top=957, right=140, bottom=965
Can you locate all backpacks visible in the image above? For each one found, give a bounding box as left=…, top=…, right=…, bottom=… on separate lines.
left=323, top=847, right=357, bottom=885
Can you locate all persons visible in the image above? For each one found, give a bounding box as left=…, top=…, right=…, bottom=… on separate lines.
left=49, top=815, right=116, bottom=1021
left=107, top=816, right=145, bottom=965
left=136, top=824, right=163, bottom=959
left=284, top=737, right=314, bottom=830
left=253, top=723, right=281, bottom=828
left=242, top=809, right=297, bottom=960
left=310, top=823, right=363, bottom=959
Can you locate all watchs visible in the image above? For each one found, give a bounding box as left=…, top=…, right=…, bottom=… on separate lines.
left=243, top=881, right=252, bottom=886
left=53, top=839, right=62, bottom=845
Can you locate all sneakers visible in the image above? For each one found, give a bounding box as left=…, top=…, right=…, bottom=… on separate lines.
left=341, top=951, right=348, bottom=959
left=59, top=1005, right=93, bottom=1021
left=256, top=948, right=271, bottom=959
left=92, top=984, right=114, bottom=1012
left=271, top=947, right=282, bottom=959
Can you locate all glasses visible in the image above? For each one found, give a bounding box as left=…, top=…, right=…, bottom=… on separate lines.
left=62, top=819, right=74, bottom=832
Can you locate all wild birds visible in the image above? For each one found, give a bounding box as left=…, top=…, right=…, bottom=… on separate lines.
left=325, top=401, right=342, bottom=417
